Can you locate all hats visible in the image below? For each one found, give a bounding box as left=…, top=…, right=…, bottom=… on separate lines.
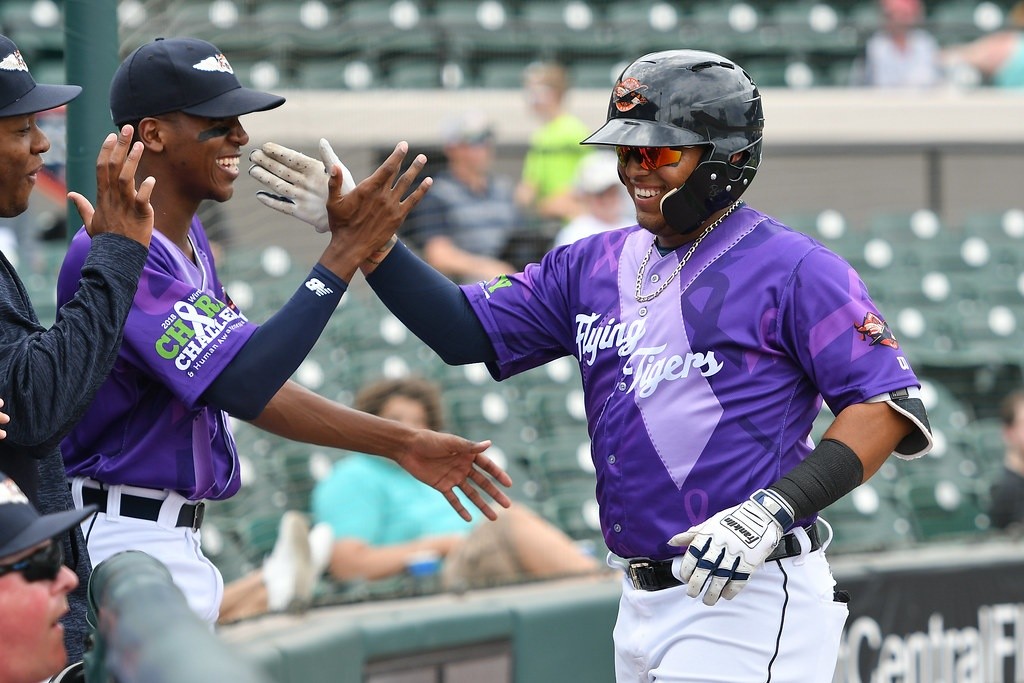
left=109, top=37, right=286, bottom=125
left=0, top=35, right=82, bottom=117
left=578, top=152, right=621, bottom=194
left=0, top=471, right=99, bottom=557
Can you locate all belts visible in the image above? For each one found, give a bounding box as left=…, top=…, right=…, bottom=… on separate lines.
left=627, top=522, right=822, bottom=592
left=67, top=482, right=205, bottom=534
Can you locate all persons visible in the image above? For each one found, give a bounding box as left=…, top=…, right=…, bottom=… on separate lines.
left=409, top=113, right=519, bottom=283
left=512, top=58, right=595, bottom=237
left=57, top=39, right=513, bottom=634
left=0, top=36, right=156, bottom=683
left=556, top=150, right=637, bottom=244
left=310, top=374, right=611, bottom=591
left=0, top=471, right=99, bottom=683
left=949, top=0, right=1024, bottom=86
left=867, top=0, right=940, bottom=87
left=987, top=387, right=1024, bottom=541
left=249, top=49, right=932, bottom=683
left=215, top=508, right=332, bottom=622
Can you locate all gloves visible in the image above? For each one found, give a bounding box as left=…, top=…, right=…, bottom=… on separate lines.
left=666, top=488, right=796, bottom=606
left=248, top=138, right=357, bottom=234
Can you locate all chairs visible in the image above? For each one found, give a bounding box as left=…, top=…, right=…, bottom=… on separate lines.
left=0, top=0, right=1024, bottom=594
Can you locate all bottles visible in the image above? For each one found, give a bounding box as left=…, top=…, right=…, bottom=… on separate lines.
left=407, top=558, right=442, bottom=596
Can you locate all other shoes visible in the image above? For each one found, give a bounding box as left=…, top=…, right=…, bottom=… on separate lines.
left=263, top=511, right=312, bottom=614
left=307, top=522, right=332, bottom=590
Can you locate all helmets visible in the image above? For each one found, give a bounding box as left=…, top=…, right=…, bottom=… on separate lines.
left=579, top=49, right=765, bottom=235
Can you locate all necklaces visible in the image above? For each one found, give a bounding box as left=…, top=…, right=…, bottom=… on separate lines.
left=635, top=200, right=742, bottom=302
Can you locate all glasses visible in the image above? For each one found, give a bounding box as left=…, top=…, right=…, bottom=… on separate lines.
left=0, top=539, right=65, bottom=581
left=615, top=144, right=712, bottom=170
